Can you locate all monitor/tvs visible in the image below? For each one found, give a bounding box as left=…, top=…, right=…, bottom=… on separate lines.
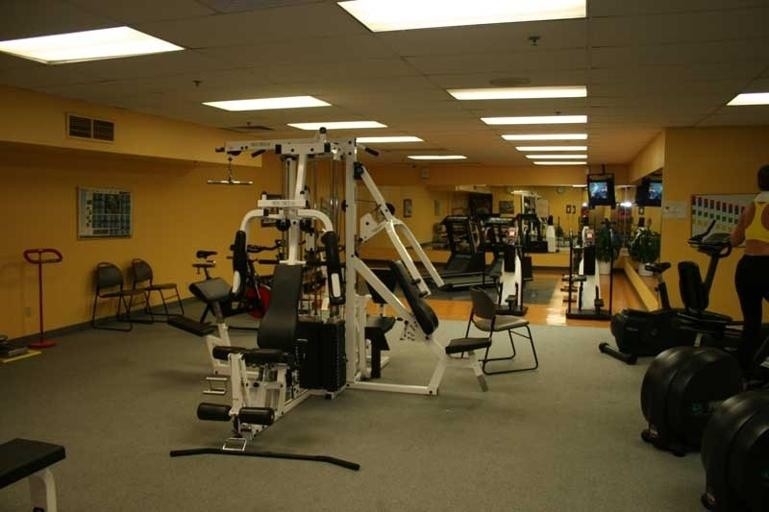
left=587, top=179, right=615, bottom=206
left=635, top=176, right=663, bottom=206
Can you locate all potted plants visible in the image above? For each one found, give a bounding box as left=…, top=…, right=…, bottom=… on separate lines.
left=630, top=227, right=660, bottom=276
left=594, top=227, right=620, bottom=274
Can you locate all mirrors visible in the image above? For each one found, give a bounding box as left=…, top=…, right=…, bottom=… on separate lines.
left=322, top=182, right=643, bottom=270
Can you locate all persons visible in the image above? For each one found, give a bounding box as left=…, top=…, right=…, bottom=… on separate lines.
left=730, top=166, right=769, bottom=363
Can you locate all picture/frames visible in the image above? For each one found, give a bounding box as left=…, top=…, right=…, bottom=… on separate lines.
left=403, top=198, right=413, bottom=218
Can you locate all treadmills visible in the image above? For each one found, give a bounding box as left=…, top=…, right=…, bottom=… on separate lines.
left=418, top=214, right=488, bottom=284
left=429, top=217, right=533, bottom=292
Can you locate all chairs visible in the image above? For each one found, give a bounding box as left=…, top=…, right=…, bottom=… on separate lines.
left=124, top=257, right=184, bottom=324
left=92, top=261, right=156, bottom=332
left=461, top=284, right=539, bottom=376
